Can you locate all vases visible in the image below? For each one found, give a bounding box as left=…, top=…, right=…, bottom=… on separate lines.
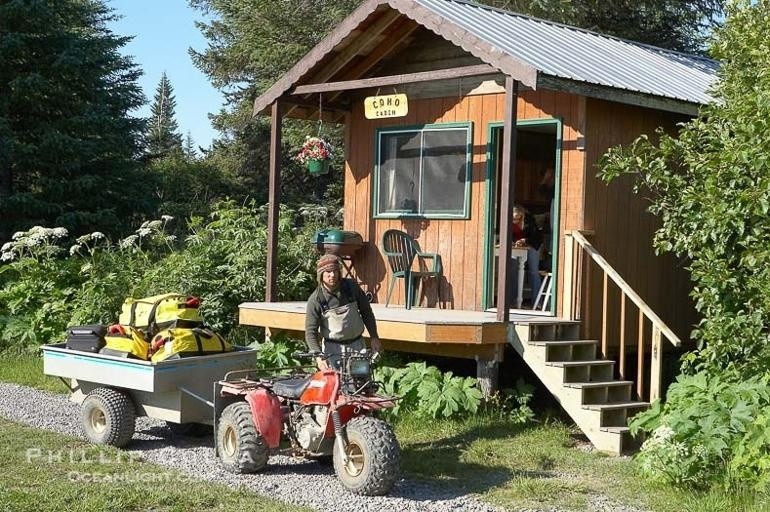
left=304, top=158, right=330, bottom=175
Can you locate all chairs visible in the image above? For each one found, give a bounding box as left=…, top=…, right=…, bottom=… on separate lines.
left=381, top=228, right=444, bottom=310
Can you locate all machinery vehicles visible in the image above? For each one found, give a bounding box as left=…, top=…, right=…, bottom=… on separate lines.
left=39, top=342, right=405, bottom=496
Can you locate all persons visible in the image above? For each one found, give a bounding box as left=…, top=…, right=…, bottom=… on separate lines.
left=512, top=203, right=543, bottom=310
left=304, top=253, right=381, bottom=370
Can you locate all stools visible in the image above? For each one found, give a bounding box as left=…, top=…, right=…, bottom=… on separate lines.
left=531, top=272, right=552, bottom=311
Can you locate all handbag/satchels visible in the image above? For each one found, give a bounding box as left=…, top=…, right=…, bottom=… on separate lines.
left=99, top=293, right=233, bottom=363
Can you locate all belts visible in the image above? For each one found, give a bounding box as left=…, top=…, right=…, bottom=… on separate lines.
left=326, top=334, right=362, bottom=344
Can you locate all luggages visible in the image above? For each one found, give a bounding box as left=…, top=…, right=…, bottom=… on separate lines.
left=65, top=325, right=107, bottom=354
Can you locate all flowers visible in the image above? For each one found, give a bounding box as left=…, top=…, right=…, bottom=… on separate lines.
left=288, top=135, right=336, bottom=166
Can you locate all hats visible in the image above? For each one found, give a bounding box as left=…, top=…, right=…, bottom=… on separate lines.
left=316, top=256, right=340, bottom=284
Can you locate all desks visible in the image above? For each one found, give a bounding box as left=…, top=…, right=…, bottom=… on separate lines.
left=493, top=244, right=531, bottom=309
